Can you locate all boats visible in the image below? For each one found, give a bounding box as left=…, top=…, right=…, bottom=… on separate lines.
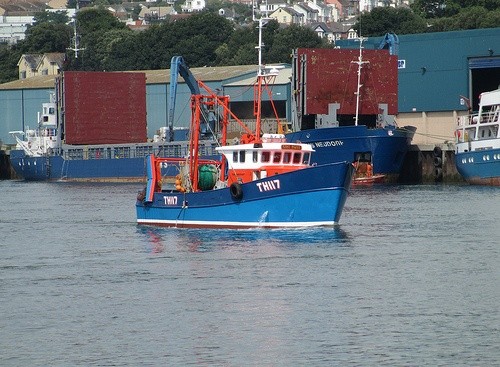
left=135, top=76, right=356, bottom=231
left=453, top=1, right=500, bottom=186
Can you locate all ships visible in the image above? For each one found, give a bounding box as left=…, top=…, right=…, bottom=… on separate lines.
left=10, top=0, right=416, bottom=187
